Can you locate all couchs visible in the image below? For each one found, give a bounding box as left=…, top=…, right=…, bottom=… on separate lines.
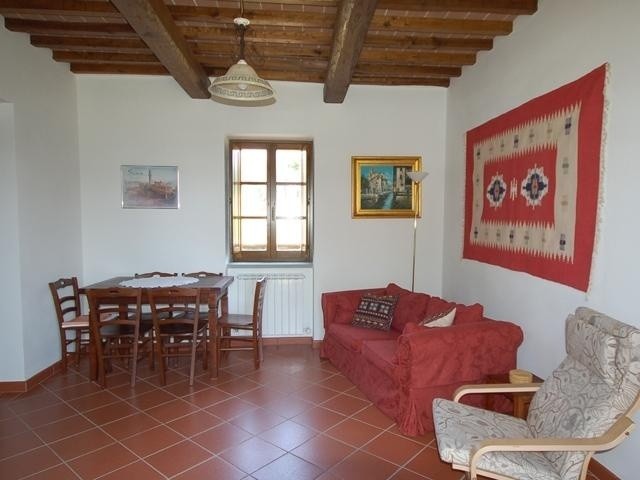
left=320, top=283, right=524, bottom=437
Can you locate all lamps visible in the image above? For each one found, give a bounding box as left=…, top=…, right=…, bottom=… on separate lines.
left=207, top=0, right=275, bottom=102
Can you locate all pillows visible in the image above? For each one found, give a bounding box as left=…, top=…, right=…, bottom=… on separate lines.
left=418, top=305, right=456, bottom=329
left=351, top=291, right=399, bottom=332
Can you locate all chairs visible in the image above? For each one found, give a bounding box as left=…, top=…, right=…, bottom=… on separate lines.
left=47, top=270, right=268, bottom=390
left=432, top=306, right=640, bottom=480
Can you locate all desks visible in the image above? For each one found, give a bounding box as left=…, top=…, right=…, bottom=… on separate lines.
left=482, top=371, right=545, bottom=420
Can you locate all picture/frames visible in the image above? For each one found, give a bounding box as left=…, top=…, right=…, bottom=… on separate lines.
left=351, top=155, right=422, bottom=218
